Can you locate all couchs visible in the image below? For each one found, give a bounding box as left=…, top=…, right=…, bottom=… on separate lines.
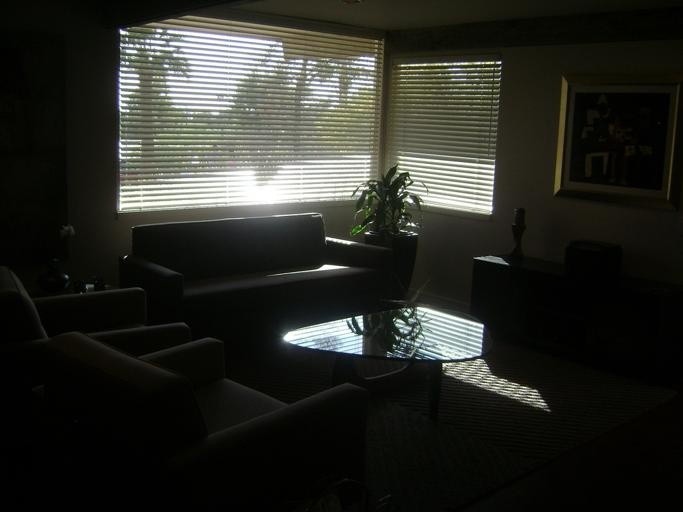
left=119, top=213, right=400, bottom=339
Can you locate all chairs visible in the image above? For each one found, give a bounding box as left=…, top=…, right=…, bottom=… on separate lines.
left=44, top=336, right=368, bottom=512
left=0, top=265, right=190, bottom=452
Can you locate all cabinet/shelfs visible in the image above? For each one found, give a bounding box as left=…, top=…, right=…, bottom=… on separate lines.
left=470, top=255, right=683, bottom=388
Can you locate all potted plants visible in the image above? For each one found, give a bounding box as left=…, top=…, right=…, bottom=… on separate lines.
left=347, top=307, right=423, bottom=358
left=349, top=164, right=425, bottom=300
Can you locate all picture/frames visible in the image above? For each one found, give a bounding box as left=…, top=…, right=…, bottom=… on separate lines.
left=552, top=71, right=682, bottom=213
